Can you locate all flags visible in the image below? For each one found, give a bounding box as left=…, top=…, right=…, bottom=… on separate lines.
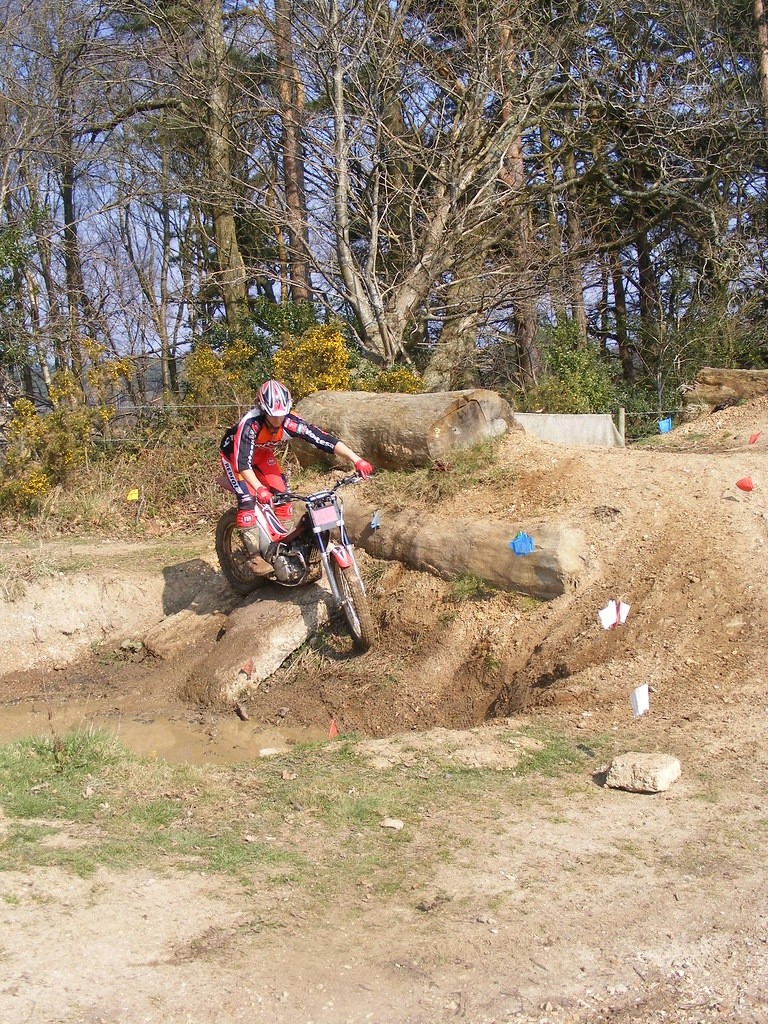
left=736, top=476, right=754, bottom=493
left=508, top=532, right=534, bottom=557
left=659, top=418, right=671, bottom=433
left=632, top=684, right=649, bottom=717
left=597, top=599, right=631, bottom=630
left=749, top=431, right=762, bottom=445
left=127, top=489, right=138, bottom=500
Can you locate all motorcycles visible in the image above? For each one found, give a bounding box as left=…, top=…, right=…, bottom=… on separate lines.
left=215, top=471, right=375, bottom=648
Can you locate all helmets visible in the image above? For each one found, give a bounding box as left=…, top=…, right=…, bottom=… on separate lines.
left=257, top=380, right=294, bottom=417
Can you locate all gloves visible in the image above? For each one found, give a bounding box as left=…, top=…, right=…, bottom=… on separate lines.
left=356, top=460, right=373, bottom=480
left=256, top=486, right=272, bottom=504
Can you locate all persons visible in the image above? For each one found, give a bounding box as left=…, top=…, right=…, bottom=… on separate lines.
left=221, top=377, right=373, bottom=583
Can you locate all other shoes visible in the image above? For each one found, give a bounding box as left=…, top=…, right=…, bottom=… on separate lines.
left=244, top=556, right=274, bottom=576
